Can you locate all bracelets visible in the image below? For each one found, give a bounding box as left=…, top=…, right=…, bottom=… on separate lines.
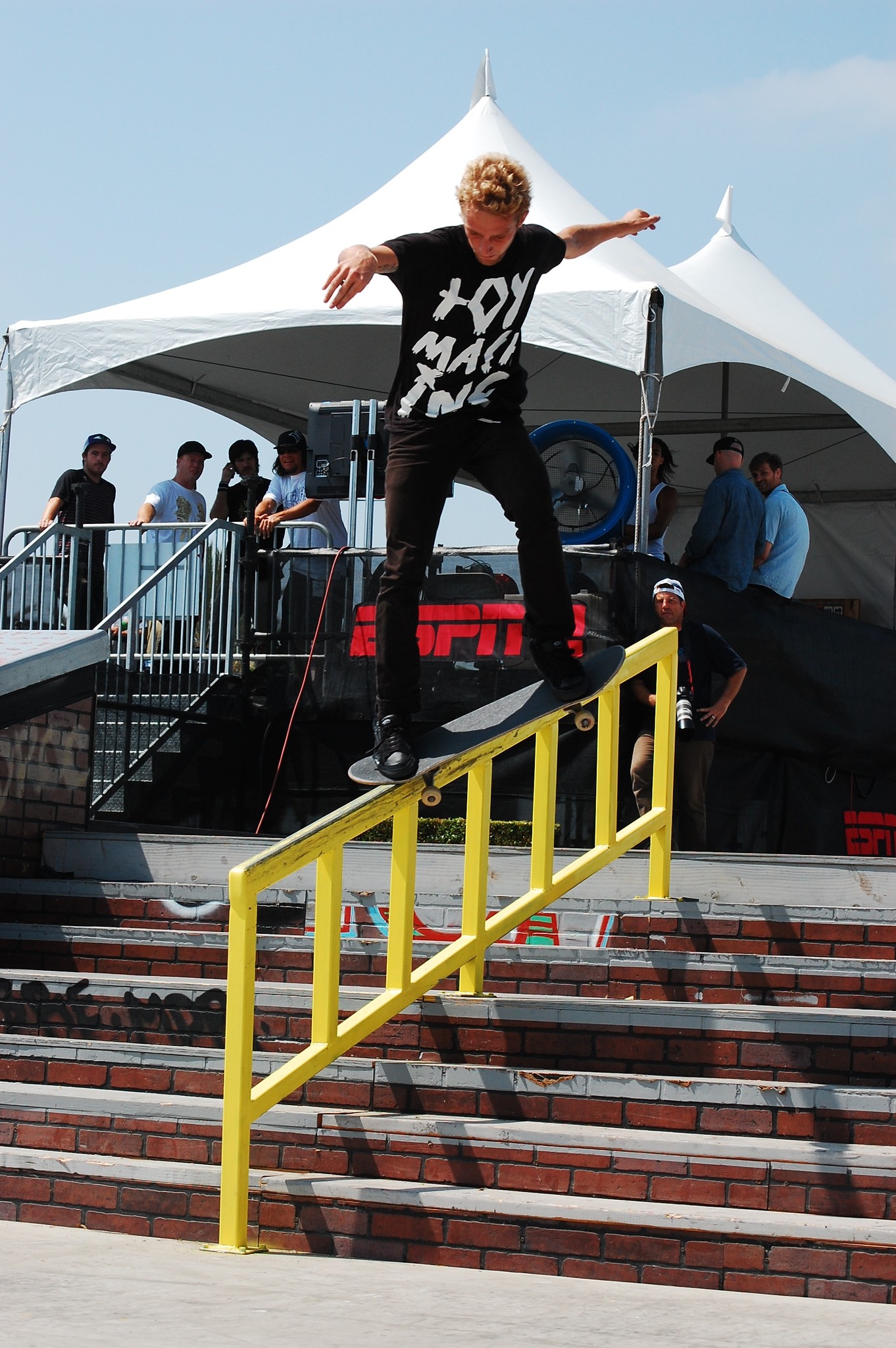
left=218, top=481, right=228, bottom=490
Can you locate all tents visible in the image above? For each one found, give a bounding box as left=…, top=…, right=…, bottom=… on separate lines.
left=0, top=48, right=896, bottom=657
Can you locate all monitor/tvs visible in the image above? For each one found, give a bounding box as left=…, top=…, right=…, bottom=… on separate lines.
left=304, top=399, right=454, bottom=500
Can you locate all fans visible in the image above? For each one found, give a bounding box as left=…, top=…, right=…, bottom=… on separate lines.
left=529, top=419, right=637, bottom=545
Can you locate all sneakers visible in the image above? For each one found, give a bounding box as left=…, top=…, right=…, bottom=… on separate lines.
left=374, top=714, right=417, bottom=777
left=529, top=639, right=587, bottom=700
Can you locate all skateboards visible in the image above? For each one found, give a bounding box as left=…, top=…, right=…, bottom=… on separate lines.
left=346, top=641, right=627, bottom=806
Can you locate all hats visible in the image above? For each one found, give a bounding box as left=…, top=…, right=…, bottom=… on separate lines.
left=653, top=578, right=684, bottom=601
left=706, top=437, right=744, bottom=465
left=273, top=430, right=307, bottom=448
left=83, top=434, right=116, bottom=452
left=177, top=441, right=212, bottom=459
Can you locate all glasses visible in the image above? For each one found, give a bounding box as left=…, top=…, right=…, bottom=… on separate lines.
left=277, top=447, right=300, bottom=455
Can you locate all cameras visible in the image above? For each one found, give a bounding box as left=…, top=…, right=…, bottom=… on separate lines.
left=676, top=686, right=694, bottom=730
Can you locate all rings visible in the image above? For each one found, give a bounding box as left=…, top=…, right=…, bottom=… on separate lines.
left=267, top=525, right=270, bottom=528
left=712, top=715, right=715, bottom=719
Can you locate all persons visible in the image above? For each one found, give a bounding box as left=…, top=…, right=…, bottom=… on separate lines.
left=37, top=152, right=810, bottom=848
